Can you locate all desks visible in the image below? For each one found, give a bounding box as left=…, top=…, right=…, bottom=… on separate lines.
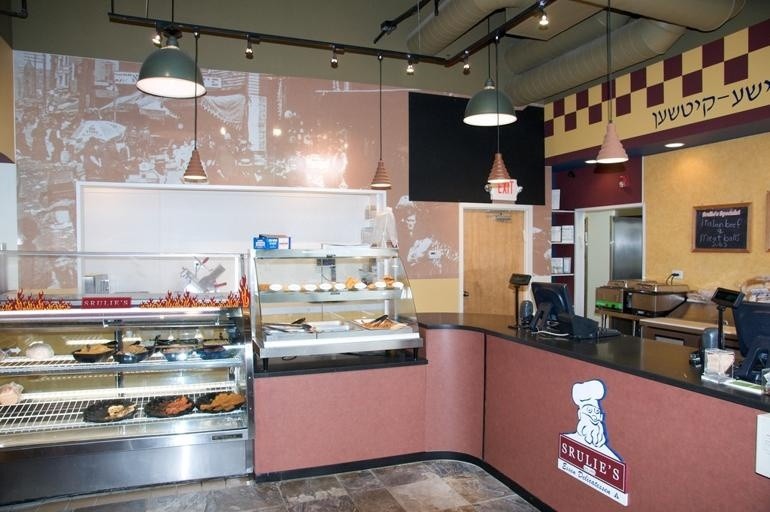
left=594, top=309, right=650, bottom=337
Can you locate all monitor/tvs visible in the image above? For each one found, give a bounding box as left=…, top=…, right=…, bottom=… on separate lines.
left=529, top=282, right=575, bottom=332
left=732, top=301, right=770, bottom=385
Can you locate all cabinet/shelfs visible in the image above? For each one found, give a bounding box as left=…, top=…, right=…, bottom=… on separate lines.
left=253, top=246, right=424, bottom=370
left=0, top=251, right=254, bottom=504
left=639, top=317, right=740, bottom=352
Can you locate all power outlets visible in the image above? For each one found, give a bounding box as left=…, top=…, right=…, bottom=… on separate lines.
left=672, top=270, right=684, bottom=280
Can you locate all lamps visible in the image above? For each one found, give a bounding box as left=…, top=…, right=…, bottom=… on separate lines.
left=463, top=15, right=518, bottom=127
left=109, top=0, right=629, bottom=188
left=135, top=0, right=207, bottom=99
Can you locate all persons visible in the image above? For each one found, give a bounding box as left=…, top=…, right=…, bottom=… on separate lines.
left=15, top=90, right=151, bottom=182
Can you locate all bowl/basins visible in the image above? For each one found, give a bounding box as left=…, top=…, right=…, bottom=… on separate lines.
left=71, top=337, right=241, bottom=363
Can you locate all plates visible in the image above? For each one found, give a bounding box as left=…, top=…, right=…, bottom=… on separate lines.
left=84, top=399, right=138, bottom=423
left=144, top=395, right=195, bottom=417
left=195, top=391, right=247, bottom=413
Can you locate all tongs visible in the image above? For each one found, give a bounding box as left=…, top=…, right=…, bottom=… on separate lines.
left=367, top=314, right=388, bottom=328
left=263, top=322, right=312, bottom=333
